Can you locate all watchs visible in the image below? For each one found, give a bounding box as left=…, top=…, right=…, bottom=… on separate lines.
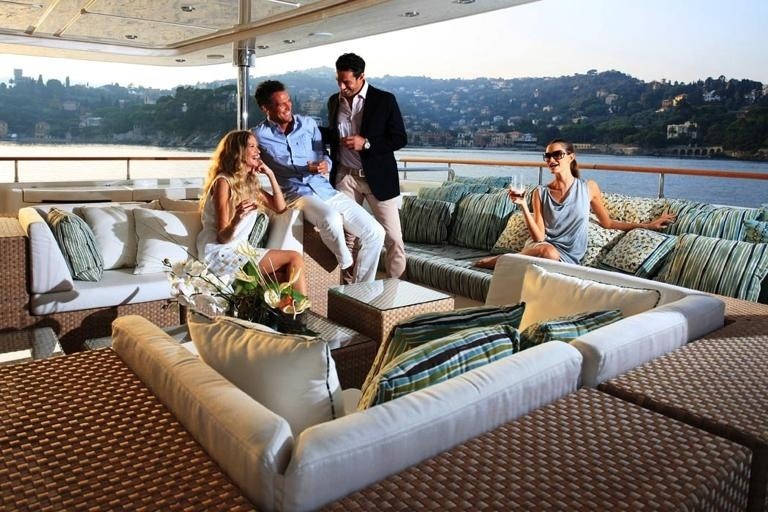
left=363, top=138, right=371, bottom=150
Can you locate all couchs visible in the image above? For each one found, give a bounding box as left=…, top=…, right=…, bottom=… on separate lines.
left=355, top=168, right=767, bottom=325
left=2, top=250, right=767, bottom=510
left=1, top=180, right=362, bottom=363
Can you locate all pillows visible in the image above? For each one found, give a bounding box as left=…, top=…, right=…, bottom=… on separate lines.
left=512, top=260, right=663, bottom=347
left=395, top=170, right=766, bottom=303
left=42, top=193, right=273, bottom=283
left=353, top=299, right=631, bottom=419
left=181, top=304, right=349, bottom=446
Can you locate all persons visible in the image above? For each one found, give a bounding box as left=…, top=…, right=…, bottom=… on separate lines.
left=196, top=129, right=308, bottom=300
left=317, top=53, right=409, bottom=281
left=250, top=81, right=386, bottom=284
left=475, top=139, right=677, bottom=269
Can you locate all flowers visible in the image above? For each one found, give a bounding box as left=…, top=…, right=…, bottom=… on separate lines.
left=130, top=206, right=326, bottom=339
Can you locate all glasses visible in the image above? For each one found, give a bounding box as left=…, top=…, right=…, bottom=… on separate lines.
left=543, top=150, right=565, bottom=162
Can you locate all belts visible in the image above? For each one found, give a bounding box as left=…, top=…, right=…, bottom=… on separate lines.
left=336, top=166, right=365, bottom=178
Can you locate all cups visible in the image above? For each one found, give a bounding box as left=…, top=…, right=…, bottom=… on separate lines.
left=511, top=169, right=530, bottom=198
left=338, top=120, right=353, bottom=139
left=307, top=151, right=323, bottom=176
left=240, top=182, right=258, bottom=211
